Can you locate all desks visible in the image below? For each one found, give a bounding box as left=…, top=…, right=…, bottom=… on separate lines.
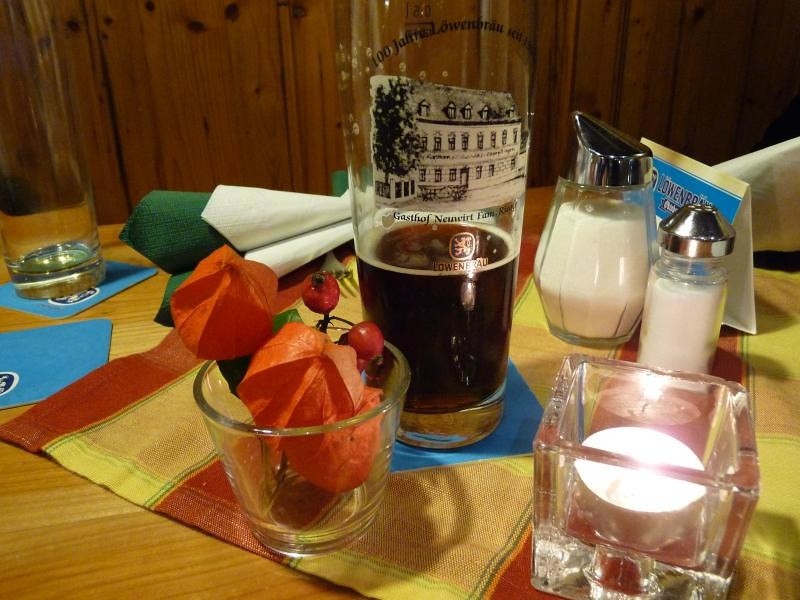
left=1, top=184, right=800, bottom=600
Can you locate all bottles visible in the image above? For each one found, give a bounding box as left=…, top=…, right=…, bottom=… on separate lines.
left=533, top=110, right=655, bottom=349
left=637, top=202, right=736, bottom=396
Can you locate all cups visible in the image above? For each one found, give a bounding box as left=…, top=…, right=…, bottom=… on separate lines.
left=336, top=0, right=540, bottom=449
left=191, top=325, right=410, bottom=555
left=532, top=355, right=758, bottom=599
left=0, top=146, right=104, bottom=298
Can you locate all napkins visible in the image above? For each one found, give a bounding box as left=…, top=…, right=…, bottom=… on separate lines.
left=711, top=137, right=800, bottom=254
left=117, top=168, right=376, bottom=329
